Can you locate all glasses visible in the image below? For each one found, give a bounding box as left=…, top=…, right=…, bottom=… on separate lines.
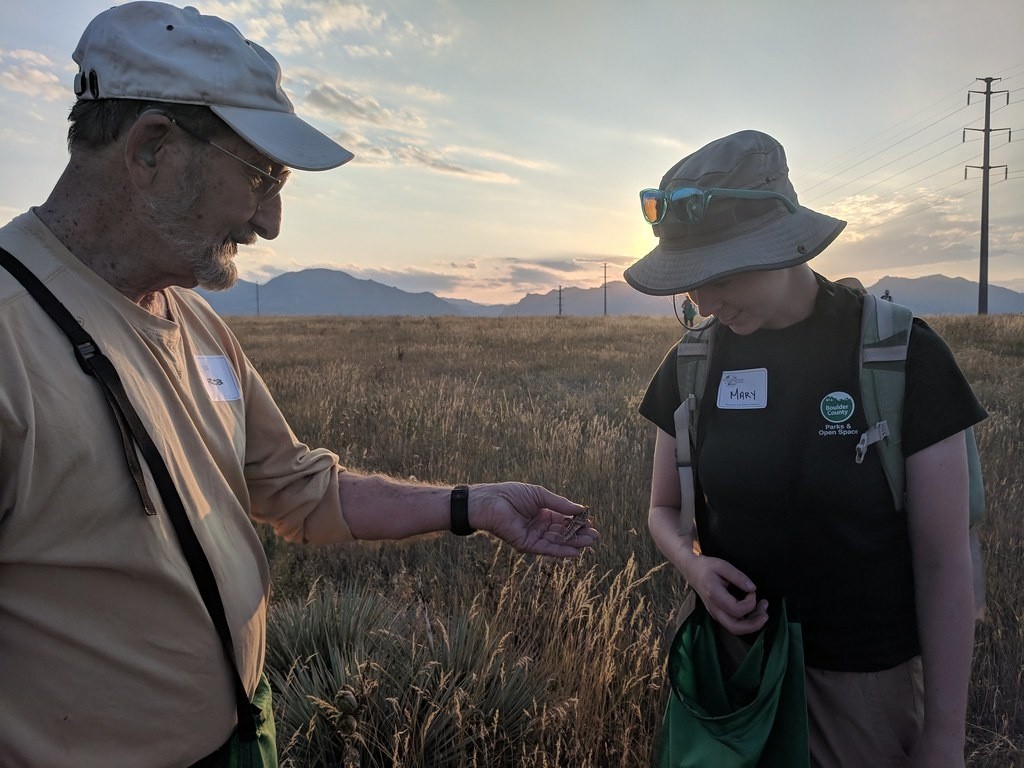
left=640, top=187, right=797, bottom=226
left=136, top=105, right=290, bottom=202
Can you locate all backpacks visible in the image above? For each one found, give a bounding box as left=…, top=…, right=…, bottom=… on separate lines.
left=673, top=278, right=985, bottom=612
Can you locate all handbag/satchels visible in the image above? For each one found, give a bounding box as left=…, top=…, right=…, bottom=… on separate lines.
left=239, top=672, right=278, bottom=768
left=650, top=593, right=809, bottom=768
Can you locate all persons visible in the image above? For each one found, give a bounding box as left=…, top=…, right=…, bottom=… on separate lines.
left=0, top=2, right=601, bottom=768
left=622, top=131, right=991, bottom=768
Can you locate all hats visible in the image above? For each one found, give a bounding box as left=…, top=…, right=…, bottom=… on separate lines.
left=72, top=1, right=354, bottom=173
left=623, top=129, right=847, bottom=296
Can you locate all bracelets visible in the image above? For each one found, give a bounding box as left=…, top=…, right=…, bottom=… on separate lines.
left=450, top=482, right=477, bottom=536
